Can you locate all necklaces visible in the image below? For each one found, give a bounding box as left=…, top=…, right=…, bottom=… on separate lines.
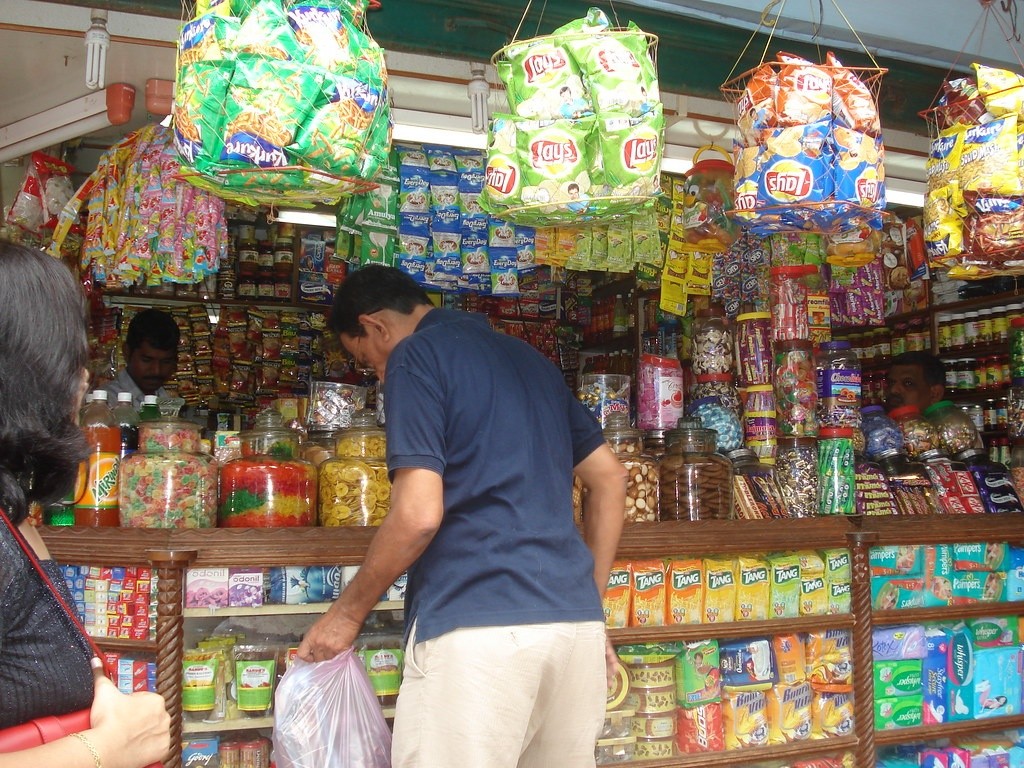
left=26, top=514, right=43, bottom=527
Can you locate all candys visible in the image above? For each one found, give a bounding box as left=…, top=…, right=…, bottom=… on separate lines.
left=708, top=226, right=883, bottom=342
left=862, top=410, right=979, bottom=460
left=578, top=319, right=859, bottom=462
left=774, top=446, right=820, bottom=518
left=219, top=438, right=314, bottom=529
left=117, top=427, right=216, bottom=529
left=39, top=124, right=229, bottom=287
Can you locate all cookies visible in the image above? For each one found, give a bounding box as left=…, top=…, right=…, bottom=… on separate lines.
left=573, top=434, right=732, bottom=523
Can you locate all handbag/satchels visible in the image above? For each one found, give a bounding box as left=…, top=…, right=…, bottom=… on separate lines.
left=0, top=506, right=164, bottom=768
left=273, top=642, right=393, bottom=768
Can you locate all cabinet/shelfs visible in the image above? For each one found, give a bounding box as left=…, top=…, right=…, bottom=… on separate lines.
left=0, top=273, right=1024, bottom=768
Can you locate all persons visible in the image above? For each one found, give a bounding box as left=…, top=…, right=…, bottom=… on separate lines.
left=0, top=237, right=171, bottom=768
left=986, top=542, right=1001, bottom=569
left=296, top=263, right=629, bottom=768
left=880, top=583, right=897, bottom=609
left=97, top=309, right=181, bottom=411
left=930, top=576, right=951, bottom=599
left=985, top=572, right=1001, bottom=601
left=897, top=545, right=915, bottom=567
left=686, top=650, right=703, bottom=672
left=886, top=351, right=947, bottom=414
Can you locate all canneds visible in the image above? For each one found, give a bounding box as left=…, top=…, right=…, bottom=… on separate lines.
left=217, top=221, right=293, bottom=302
left=938, top=302, right=1024, bottom=433
left=831, top=317, right=933, bottom=407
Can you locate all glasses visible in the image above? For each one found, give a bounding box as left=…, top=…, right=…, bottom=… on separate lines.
left=354, top=307, right=383, bottom=376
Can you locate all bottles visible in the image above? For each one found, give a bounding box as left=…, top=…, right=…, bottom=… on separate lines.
left=24, top=388, right=391, bottom=529
left=571, top=264, right=1024, bottom=525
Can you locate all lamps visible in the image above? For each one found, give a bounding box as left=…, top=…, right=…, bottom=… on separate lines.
left=143, top=78, right=492, bottom=150
left=266, top=206, right=336, bottom=228
left=659, top=141, right=735, bottom=177
left=0, top=82, right=136, bottom=163
left=885, top=176, right=926, bottom=207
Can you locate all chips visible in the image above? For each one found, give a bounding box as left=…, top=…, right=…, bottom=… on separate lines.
left=521, top=170, right=660, bottom=212
left=731, top=96, right=885, bottom=186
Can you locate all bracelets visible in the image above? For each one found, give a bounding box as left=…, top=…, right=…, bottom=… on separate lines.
left=69, top=732, right=102, bottom=768
